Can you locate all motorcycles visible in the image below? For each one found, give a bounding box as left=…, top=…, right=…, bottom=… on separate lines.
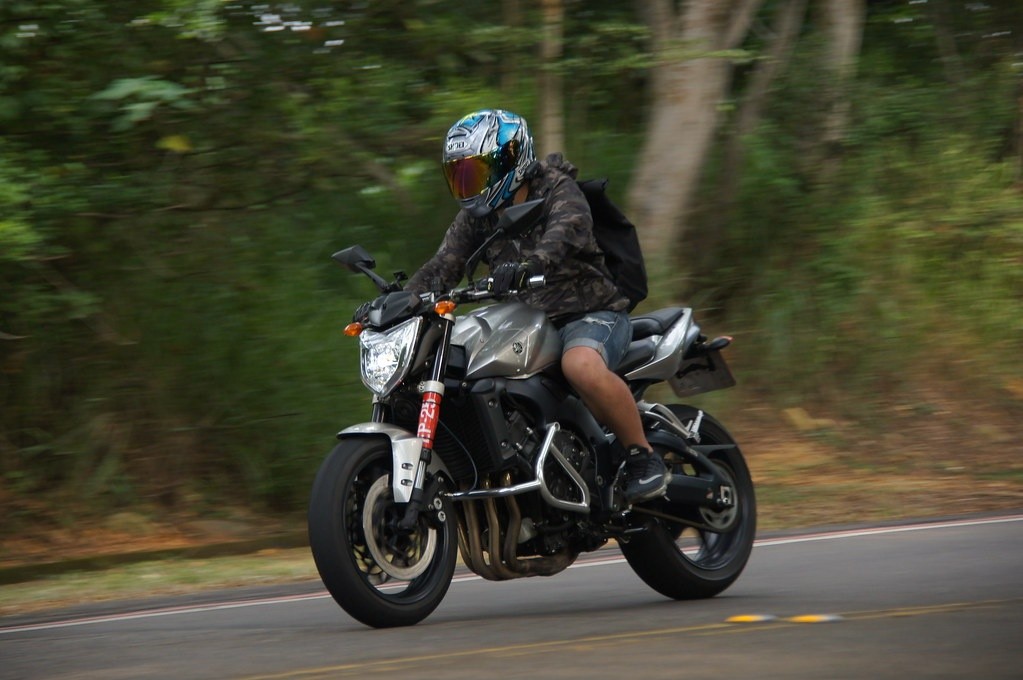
left=307, top=194, right=757, bottom=628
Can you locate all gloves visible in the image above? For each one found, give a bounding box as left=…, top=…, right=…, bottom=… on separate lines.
left=487, top=260, right=539, bottom=297
left=351, top=301, right=372, bottom=324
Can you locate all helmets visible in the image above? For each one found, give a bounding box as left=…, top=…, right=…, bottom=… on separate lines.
left=443, top=108, right=537, bottom=218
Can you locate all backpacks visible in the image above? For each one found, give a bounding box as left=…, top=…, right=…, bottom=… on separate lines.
left=576, top=177, right=649, bottom=313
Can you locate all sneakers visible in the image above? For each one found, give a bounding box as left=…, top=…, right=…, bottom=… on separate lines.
left=623, top=443, right=674, bottom=504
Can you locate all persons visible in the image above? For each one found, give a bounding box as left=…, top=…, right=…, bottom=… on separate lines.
left=352, top=111, right=674, bottom=504
